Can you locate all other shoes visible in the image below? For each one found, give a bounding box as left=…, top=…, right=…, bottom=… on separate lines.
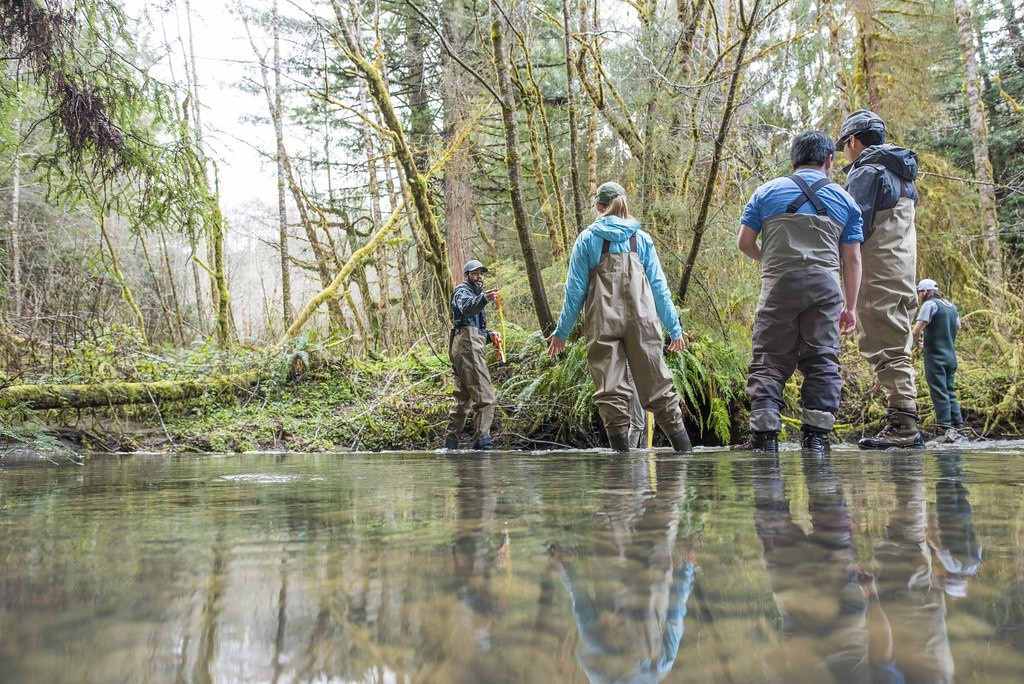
left=734, top=432, right=778, bottom=453
left=800, top=431, right=830, bottom=452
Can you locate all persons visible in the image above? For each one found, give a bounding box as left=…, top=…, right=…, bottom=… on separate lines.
left=449, top=458, right=981, bottom=684
left=834, top=109, right=926, bottom=451
left=444, top=260, right=502, bottom=450
left=911, top=278, right=966, bottom=437
left=731, top=131, right=864, bottom=454
left=545, top=182, right=692, bottom=454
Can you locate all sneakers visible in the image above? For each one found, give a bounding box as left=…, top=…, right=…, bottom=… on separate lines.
left=858, top=413, right=925, bottom=450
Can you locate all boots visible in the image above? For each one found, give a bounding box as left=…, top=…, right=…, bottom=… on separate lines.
left=666, top=428, right=692, bottom=451
left=608, top=432, right=629, bottom=452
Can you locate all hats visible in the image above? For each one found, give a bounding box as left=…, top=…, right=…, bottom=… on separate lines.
left=916, top=279, right=938, bottom=290
left=595, top=182, right=627, bottom=204
left=834, top=109, right=886, bottom=151
left=463, top=260, right=488, bottom=274
